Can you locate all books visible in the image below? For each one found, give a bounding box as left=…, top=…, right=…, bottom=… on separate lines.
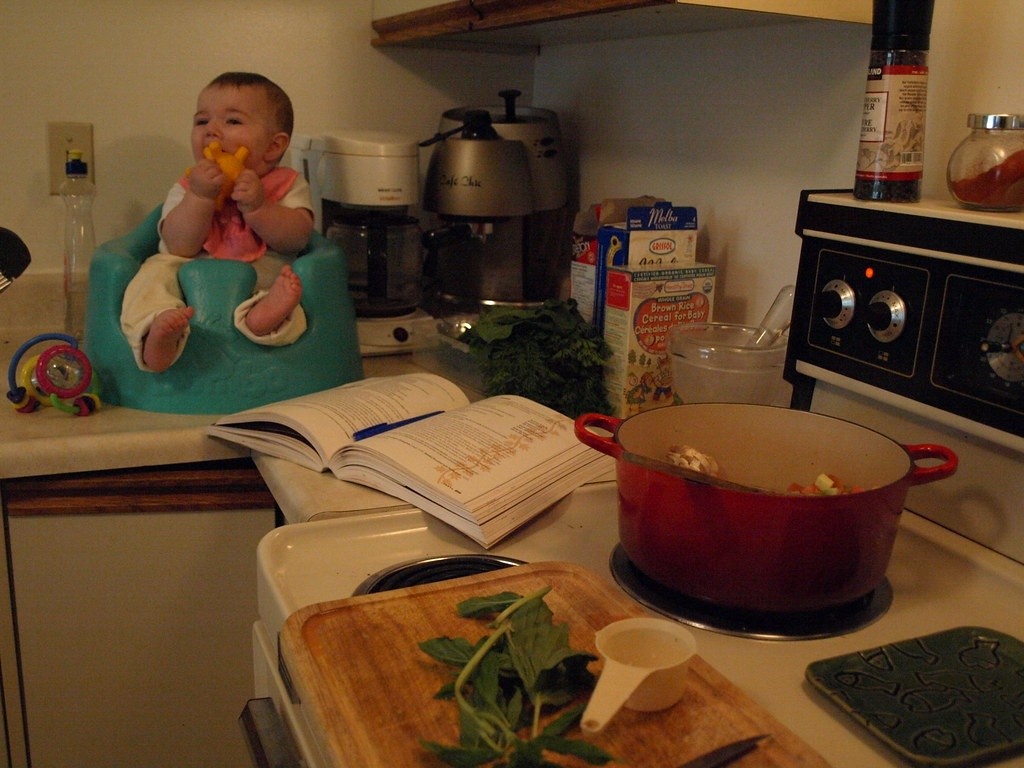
left=203, top=371, right=617, bottom=551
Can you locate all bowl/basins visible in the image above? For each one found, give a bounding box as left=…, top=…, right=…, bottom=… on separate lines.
left=665, top=320, right=789, bottom=407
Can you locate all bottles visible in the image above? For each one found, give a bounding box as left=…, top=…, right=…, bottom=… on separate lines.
left=854, top=0, right=934, bottom=202
left=946, top=114, right=1024, bottom=213
left=59, top=149, right=96, bottom=343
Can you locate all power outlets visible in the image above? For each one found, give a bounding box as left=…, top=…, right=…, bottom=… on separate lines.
left=46, top=121, right=94, bottom=195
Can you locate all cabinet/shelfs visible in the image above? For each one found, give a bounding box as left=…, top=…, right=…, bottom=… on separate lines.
left=0, top=491, right=282, bottom=768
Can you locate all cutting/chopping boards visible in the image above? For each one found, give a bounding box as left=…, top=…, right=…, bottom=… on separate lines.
left=279, top=560, right=831, bottom=768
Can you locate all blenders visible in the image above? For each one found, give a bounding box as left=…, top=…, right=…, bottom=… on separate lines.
left=420, top=110, right=532, bottom=336
left=439, top=89, right=570, bottom=306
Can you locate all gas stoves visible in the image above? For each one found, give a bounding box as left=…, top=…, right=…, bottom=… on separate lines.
left=242, top=188, right=1024, bottom=768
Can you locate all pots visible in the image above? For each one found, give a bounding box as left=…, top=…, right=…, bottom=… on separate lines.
left=573, top=404, right=957, bottom=613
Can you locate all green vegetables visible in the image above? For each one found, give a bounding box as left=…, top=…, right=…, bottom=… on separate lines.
left=415, top=582, right=613, bottom=768
left=437, top=298, right=618, bottom=420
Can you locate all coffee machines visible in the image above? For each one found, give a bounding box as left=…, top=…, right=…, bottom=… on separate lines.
left=291, top=130, right=439, bottom=357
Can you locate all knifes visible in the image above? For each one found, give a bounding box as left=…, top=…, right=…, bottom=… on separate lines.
left=677, top=733, right=772, bottom=768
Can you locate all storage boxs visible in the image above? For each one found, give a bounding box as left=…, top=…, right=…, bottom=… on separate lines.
left=593, top=195, right=699, bottom=339
left=569, top=203, right=601, bottom=329
left=601, top=261, right=716, bottom=419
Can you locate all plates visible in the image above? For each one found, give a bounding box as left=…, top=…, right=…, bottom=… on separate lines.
left=804, top=626, right=1024, bottom=768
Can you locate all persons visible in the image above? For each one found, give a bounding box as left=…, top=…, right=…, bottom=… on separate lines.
left=119, top=69, right=316, bottom=374
